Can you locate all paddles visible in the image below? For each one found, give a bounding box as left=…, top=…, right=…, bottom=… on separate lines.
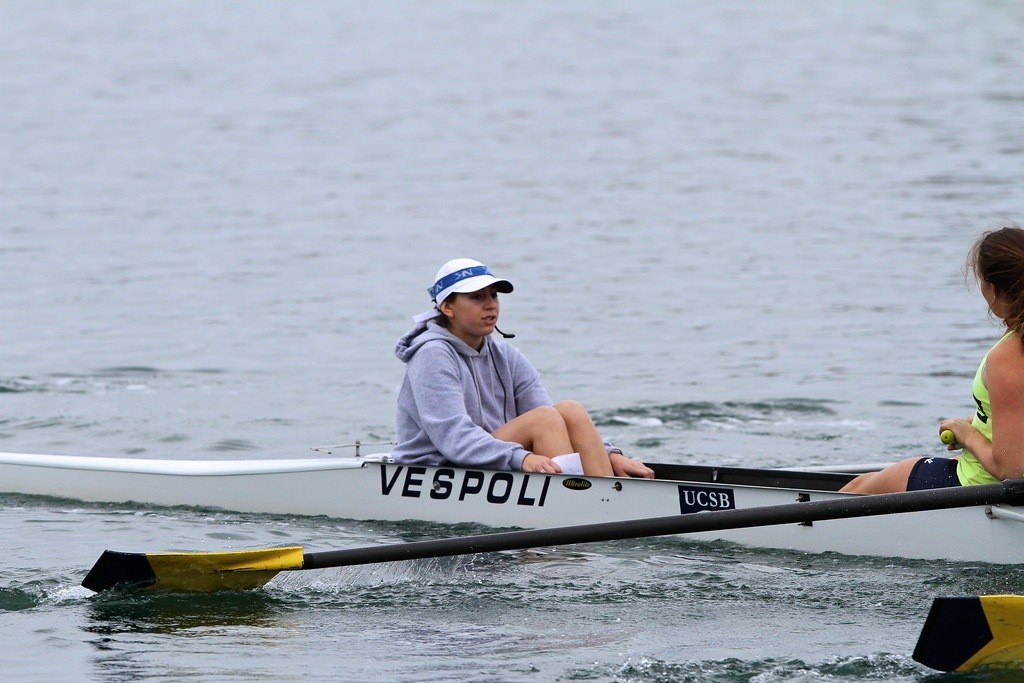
left=939, top=429, right=958, bottom=444
left=910, top=593, right=1024, bottom=674
left=77, top=476, right=1024, bottom=599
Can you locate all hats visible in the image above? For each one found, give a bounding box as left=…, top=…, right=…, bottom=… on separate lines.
left=427, top=259, right=513, bottom=310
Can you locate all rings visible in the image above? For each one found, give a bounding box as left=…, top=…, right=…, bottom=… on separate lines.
left=541, top=469, right=545, bottom=471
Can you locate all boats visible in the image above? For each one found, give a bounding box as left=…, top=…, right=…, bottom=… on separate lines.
left=0, top=445, right=1024, bottom=570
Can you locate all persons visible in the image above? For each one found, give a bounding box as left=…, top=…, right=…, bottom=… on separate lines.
left=835, top=229, right=1024, bottom=495
left=390, top=258, right=655, bottom=482
